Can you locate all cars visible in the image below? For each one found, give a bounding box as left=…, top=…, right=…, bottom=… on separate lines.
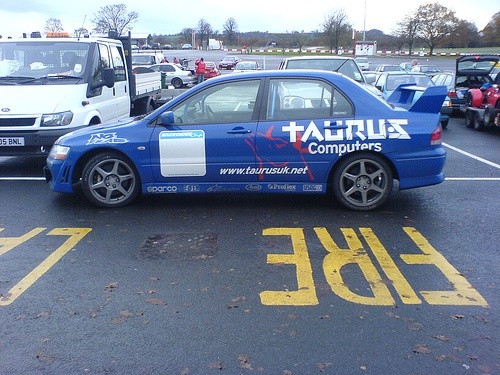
left=276, top=54, right=500, bottom=133
left=231, top=61, right=262, bottom=71
left=147, top=62, right=198, bottom=89
left=219, top=55, right=242, bottom=70
left=163, top=44, right=173, bottom=50
left=130, top=43, right=161, bottom=50
left=42, top=68, right=450, bottom=213
left=195, top=62, right=223, bottom=80
left=182, top=44, right=192, bottom=50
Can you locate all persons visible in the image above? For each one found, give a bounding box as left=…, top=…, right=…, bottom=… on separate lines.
left=161, top=57, right=169, bottom=63
left=195, top=58, right=206, bottom=84
left=411, top=58, right=419, bottom=72
left=174, top=58, right=177, bottom=63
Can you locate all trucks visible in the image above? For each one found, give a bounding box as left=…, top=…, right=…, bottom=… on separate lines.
left=129, top=49, right=164, bottom=66
left=0, top=30, right=163, bottom=159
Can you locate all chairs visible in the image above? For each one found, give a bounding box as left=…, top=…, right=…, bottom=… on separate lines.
left=27, top=49, right=84, bottom=72
